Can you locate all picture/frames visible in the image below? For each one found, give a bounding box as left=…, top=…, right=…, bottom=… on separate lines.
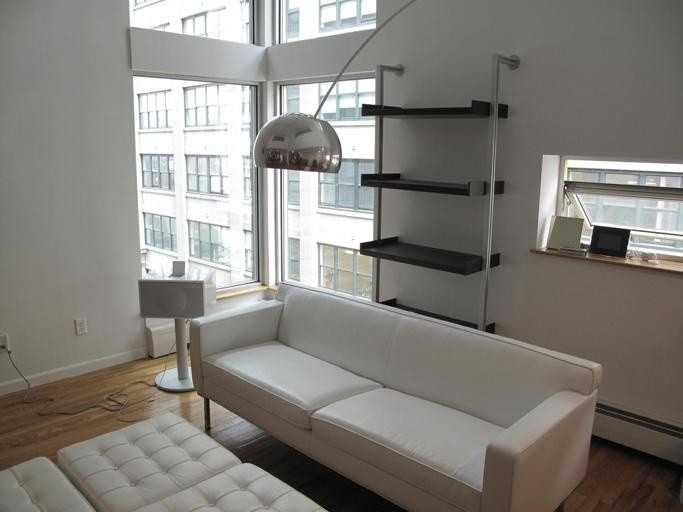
left=587, top=224, right=630, bottom=260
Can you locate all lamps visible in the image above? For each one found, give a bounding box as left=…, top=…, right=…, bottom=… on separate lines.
left=248, top=111, right=339, bottom=175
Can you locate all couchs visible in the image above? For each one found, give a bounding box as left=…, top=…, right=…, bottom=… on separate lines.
left=190, top=279, right=607, bottom=511
left=0, top=412, right=330, bottom=512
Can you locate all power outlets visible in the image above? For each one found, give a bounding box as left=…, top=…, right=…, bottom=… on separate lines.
left=75, top=316, right=87, bottom=335
left=0, top=333, right=10, bottom=352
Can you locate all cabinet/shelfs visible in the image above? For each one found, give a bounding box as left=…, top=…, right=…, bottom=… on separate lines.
left=357, top=52, right=518, bottom=337
